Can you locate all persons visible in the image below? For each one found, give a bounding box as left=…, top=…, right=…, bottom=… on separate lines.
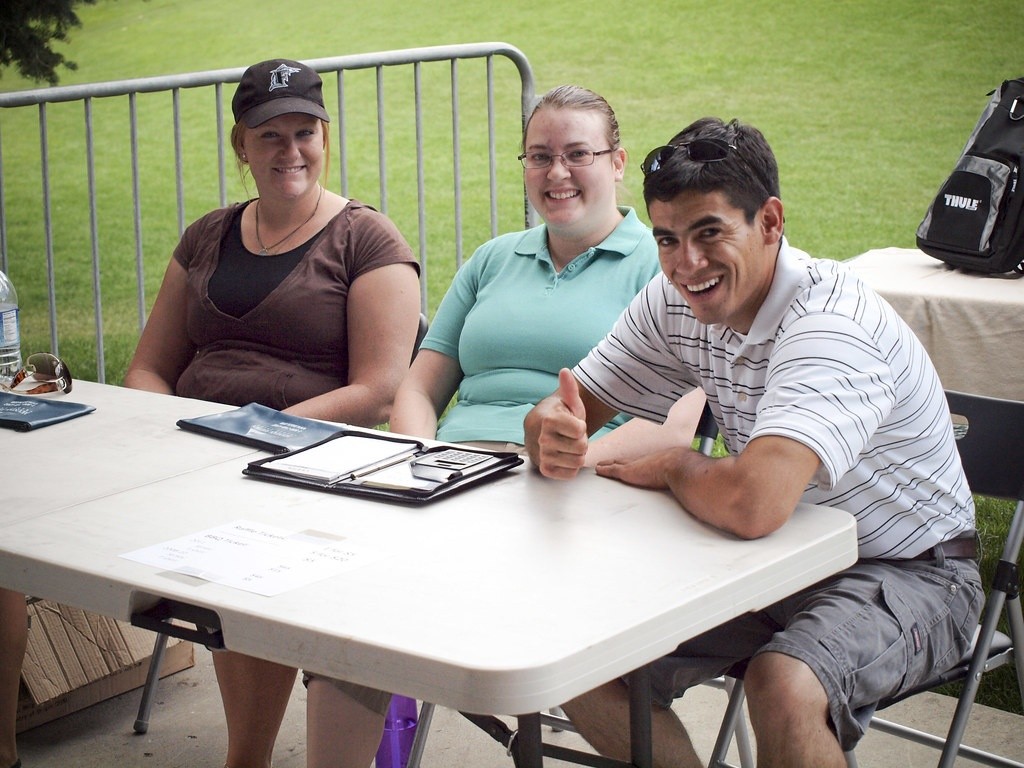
left=0, top=58, right=419, bottom=767
left=299, top=86, right=664, bottom=767
left=521, top=116, right=988, bottom=768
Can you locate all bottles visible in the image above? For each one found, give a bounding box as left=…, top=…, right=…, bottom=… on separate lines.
left=0, top=270, right=22, bottom=389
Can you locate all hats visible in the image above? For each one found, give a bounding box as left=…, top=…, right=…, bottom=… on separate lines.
left=232, top=59, right=330, bottom=128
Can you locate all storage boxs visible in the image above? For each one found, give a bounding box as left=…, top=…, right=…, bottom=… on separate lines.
left=14, top=598, right=198, bottom=736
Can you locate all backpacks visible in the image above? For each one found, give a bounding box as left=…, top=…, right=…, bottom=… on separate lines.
left=915, top=76, right=1024, bottom=276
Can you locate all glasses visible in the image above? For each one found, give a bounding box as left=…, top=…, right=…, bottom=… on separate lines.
left=0, top=353, right=72, bottom=396
left=640, top=137, right=754, bottom=176
left=518, top=147, right=614, bottom=169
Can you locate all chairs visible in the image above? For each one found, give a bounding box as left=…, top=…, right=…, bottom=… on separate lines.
left=706, top=391, right=1024, bottom=768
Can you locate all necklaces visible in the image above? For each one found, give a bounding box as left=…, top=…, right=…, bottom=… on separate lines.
left=255, top=185, right=322, bottom=255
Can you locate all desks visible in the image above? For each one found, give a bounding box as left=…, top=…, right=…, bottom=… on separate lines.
left=0, top=371, right=858, bottom=768
left=840, top=246, right=1024, bottom=501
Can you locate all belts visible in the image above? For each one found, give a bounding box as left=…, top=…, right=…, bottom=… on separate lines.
left=913, top=536, right=978, bottom=559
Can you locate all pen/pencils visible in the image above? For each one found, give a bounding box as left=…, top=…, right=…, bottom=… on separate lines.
left=350, top=453, right=416, bottom=481
